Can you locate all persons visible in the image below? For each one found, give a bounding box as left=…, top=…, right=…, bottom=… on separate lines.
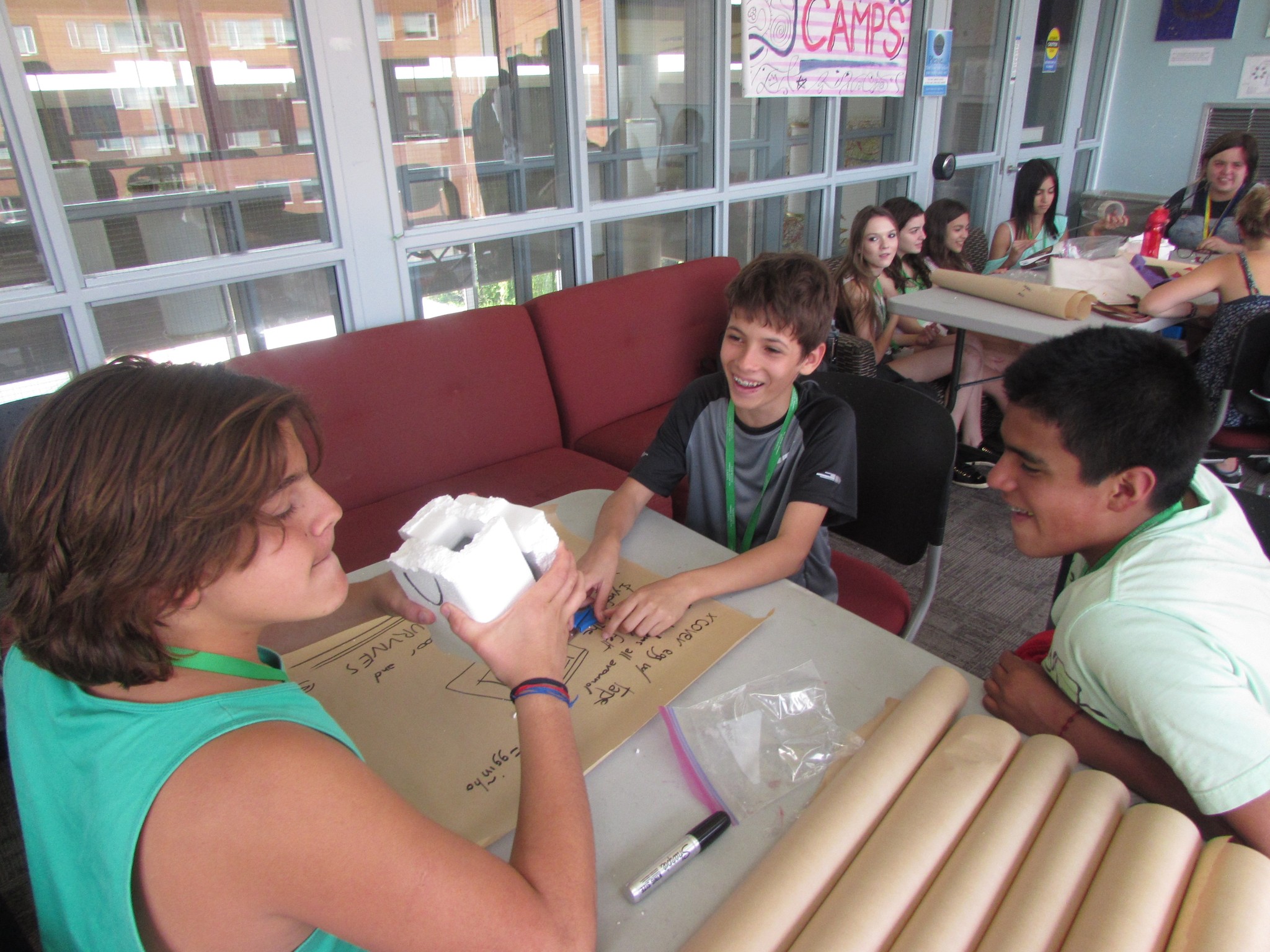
left=1136, top=180, right=1270, bottom=492
left=466, top=21, right=728, bottom=247
left=983, top=323, right=1270, bottom=855
left=1, top=354, right=599, bottom=952
left=1145, top=127, right=1267, bottom=255
left=568, top=252, right=857, bottom=644
left=821, top=194, right=1031, bottom=489
left=982, top=158, right=1128, bottom=275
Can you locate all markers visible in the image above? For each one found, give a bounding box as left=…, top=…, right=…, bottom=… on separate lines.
left=623, top=811, right=730, bottom=903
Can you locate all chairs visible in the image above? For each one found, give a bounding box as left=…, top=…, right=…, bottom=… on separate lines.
left=1181, top=296, right=1270, bottom=450
left=774, top=370, right=956, bottom=642
left=1044, top=445, right=1270, bottom=691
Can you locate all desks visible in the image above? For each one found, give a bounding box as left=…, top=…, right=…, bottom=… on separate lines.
left=251, top=488, right=1270, bottom=952
left=888, top=236, right=1232, bottom=416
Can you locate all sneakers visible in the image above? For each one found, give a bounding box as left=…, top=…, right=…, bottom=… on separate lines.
left=1202, top=463, right=1243, bottom=489
left=952, top=459, right=989, bottom=489
left=958, top=439, right=1003, bottom=467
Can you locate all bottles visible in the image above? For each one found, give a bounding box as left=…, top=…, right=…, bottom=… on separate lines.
left=824, top=319, right=839, bottom=370
left=1140, top=208, right=1169, bottom=258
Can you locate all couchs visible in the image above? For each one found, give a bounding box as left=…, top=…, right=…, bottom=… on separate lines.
left=0, top=304, right=673, bottom=619
left=812, top=225, right=1002, bottom=407
left=527, top=256, right=742, bottom=528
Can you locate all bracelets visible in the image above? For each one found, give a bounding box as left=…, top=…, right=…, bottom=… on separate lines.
left=509, top=676, right=579, bottom=709
left=1057, top=708, right=1083, bottom=736
